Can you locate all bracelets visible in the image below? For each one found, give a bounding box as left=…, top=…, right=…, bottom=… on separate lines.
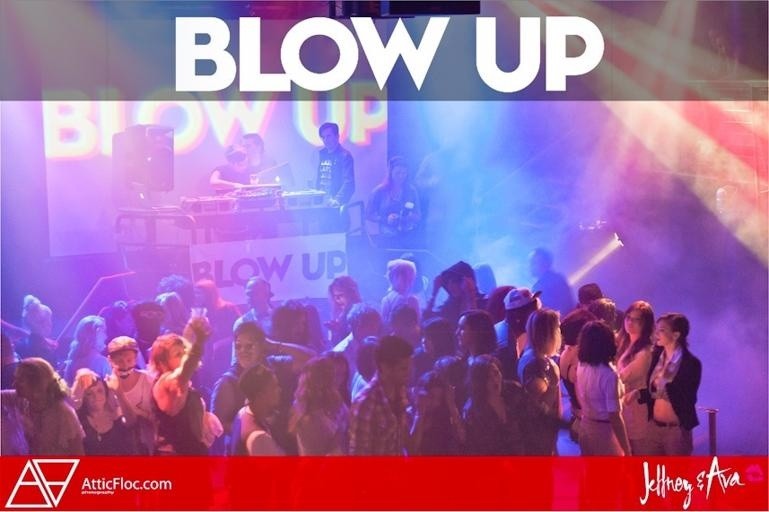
left=184, top=349, right=199, bottom=357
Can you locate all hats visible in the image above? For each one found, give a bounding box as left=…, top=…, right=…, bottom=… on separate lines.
left=239, top=361, right=272, bottom=395
left=107, top=335, right=138, bottom=354
left=504, top=288, right=542, bottom=310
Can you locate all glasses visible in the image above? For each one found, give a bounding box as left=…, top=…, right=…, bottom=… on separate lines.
left=234, top=342, right=254, bottom=349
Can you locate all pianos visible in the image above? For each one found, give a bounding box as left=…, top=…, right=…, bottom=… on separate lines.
left=176, top=183, right=341, bottom=228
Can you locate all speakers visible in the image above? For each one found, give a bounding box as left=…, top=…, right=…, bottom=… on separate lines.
left=121, top=125, right=173, bottom=192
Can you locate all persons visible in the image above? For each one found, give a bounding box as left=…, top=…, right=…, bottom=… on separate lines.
left=575, top=320, right=632, bottom=457
left=350, top=335, right=378, bottom=399
left=473, top=263, right=497, bottom=297
left=315, top=122, right=356, bottom=208
left=380, top=259, right=422, bottom=326
left=494, top=286, right=542, bottom=372
left=323, top=275, right=360, bottom=345
left=241, top=132, right=281, bottom=185
left=622, top=312, right=703, bottom=456
left=148, top=306, right=210, bottom=458
left=559, top=309, right=598, bottom=444
left=455, top=309, right=507, bottom=456
left=193, top=281, right=239, bottom=339
left=230, top=277, right=276, bottom=365
left=0, top=356, right=85, bottom=458
left=208, top=145, right=249, bottom=195
left=577, top=283, right=604, bottom=308
left=269, top=301, right=316, bottom=349
left=157, top=290, right=188, bottom=336
left=616, top=300, right=656, bottom=456
left=364, top=156, right=422, bottom=250
left=487, top=284, right=514, bottom=317
left=287, top=355, right=348, bottom=458
left=345, top=303, right=380, bottom=350
left=101, top=335, right=154, bottom=457
left=225, top=363, right=286, bottom=458
left=1, top=293, right=63, bottom=370
left=415, top=317, right=466, bottom=375
left=64, top=314, right=111, bottom=387
left=410, top=368, right=464, bottom=456
left=588, top=298, right=618, bottom=333
left=98, top=299, right=146, bottom=372
left=74, top=367, right=131, bottom=457
left=126, top=294, right=162, bottom=352
left=157, top=275, right=194, bottom=312
left=343, top=335, right=413, bottom=458
left=210, top=321, right=318, bottom=457
left=516, top=307, right=582, bottom=456
left=525, top=249, right=573, bottom=311
left=422, top=261, right=485, bottom=320
left=389, top=303, right=420, bottom=349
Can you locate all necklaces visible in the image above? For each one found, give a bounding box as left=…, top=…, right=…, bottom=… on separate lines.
left=92, top=420, right=108, bottom=443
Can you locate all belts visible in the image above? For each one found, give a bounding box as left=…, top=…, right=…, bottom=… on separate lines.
left=598, top=419, right=611, bottom=424
left=652, top=418, right=680, bottom=427
